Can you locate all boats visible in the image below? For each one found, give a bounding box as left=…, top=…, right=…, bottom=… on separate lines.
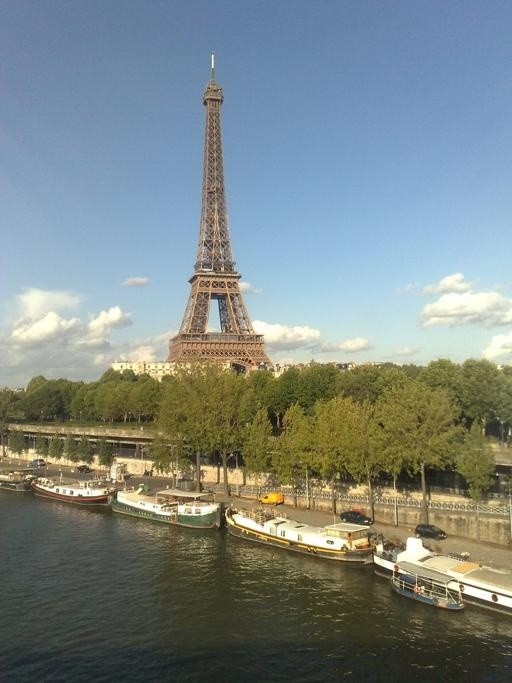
left=374, top=536, right=512, bottom=610
left=0, top=469, right=220, bottom=529
left=225, top=504, right=373, bottom=565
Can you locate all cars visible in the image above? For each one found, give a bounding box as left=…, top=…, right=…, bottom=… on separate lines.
left=416, top=525, right=447, bottom=541
left=340, top=510, right=371, bottom=526
left=29, top=459, right=47, bottom=468
left=77, top=465, right=90, bottom=473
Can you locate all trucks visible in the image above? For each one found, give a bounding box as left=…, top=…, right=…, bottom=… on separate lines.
left=257, top=492, right=283, bottom=505
